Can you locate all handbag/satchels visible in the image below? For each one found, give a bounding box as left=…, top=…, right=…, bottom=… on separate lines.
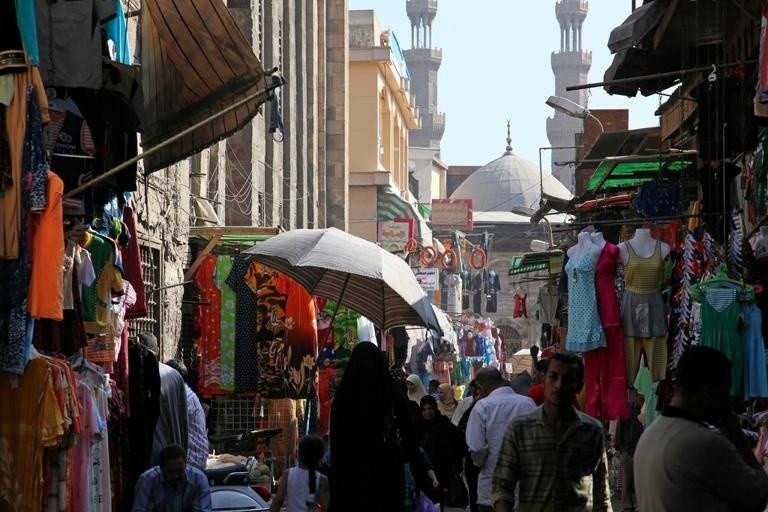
left=444, top=477, right=469, bottom=507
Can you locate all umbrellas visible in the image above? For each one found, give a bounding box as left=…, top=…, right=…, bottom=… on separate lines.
left=240, top=225, right=444, bottom=386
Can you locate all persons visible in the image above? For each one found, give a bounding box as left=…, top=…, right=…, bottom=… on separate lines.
left=563, top=232, right=607, bottom=355
left=511, top=285, right=528, bottom=317
left=443, top=270, right=502, bottom=314
left=617, top=229, right=670, bottom=337
left=589, top=232, right=623, bottom=329
